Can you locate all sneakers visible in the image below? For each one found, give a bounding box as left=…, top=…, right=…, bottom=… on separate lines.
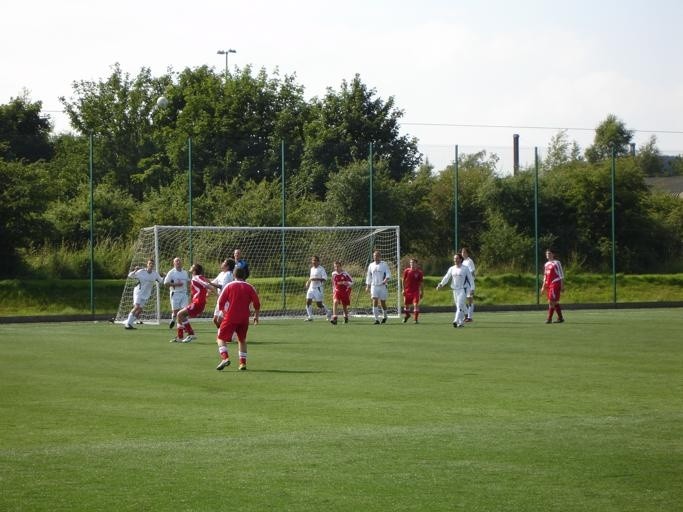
left=304, top=317, right=314, bottom=322
left=373, top=320, right=380, bottom=325
left=403, top=314, right=410, bottom=323
left=182, top=335, right=197, bottom=343
left=381, top=318, right=387, bottom=323
left=125, top=324, right=137, bottom=330
left=544, top=319, right=563, bottom=323
left=216, top=358, right=231, bottom=370
left=325, top=310, right=347, bottom=325
left=238, top=365, right=246, bottom=371
left=169, top=320, right=175, bottom=329
left=169, top=337, right=183, bottom=342
left=452, top=314, right=471, bottom=328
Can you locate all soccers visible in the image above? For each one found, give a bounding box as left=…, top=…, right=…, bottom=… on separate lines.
left=157, top=97, right=168, bottom=109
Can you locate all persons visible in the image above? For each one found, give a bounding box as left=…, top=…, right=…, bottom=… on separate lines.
left=365, top=251, right=391, bottom=324
left=232, top=250, right=249, bottom=278
left=330, top=261, right=353, bottom=325
left=401, top=258, right=424, bottom=324
left=206, top=258, right=237, bottom=344
left=124, top=260, right=167, bottom=329
left=163, top=258, right=191, bottom=333
left=461, top=247, right=475, bottom=322
left=216, top=266, right=260, bottom=370
left=170, top=264, right=215, bottom=342
left=540, top=248, right=565, bottom=323
left=435, top=253, right=475, bottom=327
left=303, top=256, right=331, bottom=322
